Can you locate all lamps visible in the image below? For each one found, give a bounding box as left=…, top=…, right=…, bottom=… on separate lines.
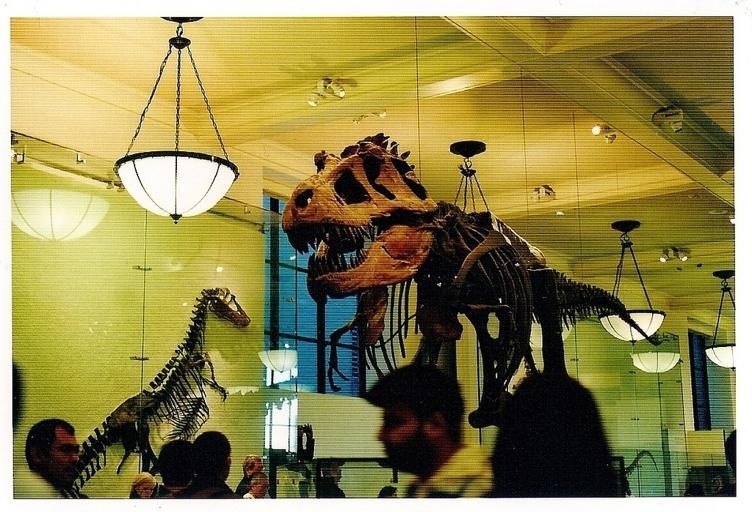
left=627, top=350, right=680, bottom=375
left=594, top=220, right=667, bottom=347
left=257, top=350, right=299, bottom=374
left=705, top=268, right=737, bottom=374
left=114, top=17, right=242, bottom=225
left=12, top=181, right=110, bottom=251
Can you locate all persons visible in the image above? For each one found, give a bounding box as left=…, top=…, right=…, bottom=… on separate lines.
left=242, top=471, right=270, bottom=499
left=25, top=416, right=85, bottom=499
left=492, top=369, right=621, bottom=497
left=173, top=430, right=242, bottom=497
left=156, top=439, right=196, bottom=497
left=235, top=454, right=265, bottom=497
left=129, top=470, right=157, bottom=498
left=712, top=429, right=736, bottom=496
left=315, top=461, right=346, bottom=498
left=360, top=361, right=495, bottom=498
